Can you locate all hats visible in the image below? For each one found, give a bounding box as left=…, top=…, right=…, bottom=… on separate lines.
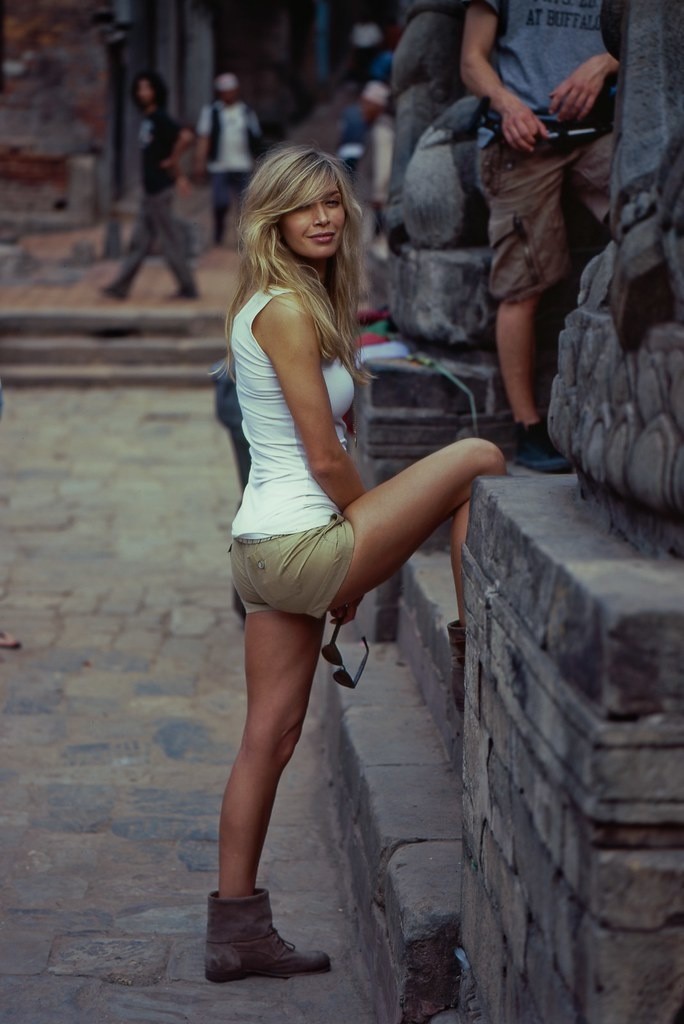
left=352, top=23, right=381, bottom=47
left=216, top=72, right=238, bottom=90
left=362, top=80, right=389, bottom=106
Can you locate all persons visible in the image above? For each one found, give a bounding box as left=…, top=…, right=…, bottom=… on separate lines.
left=337, top=97, right=368, bottom=171
left=461, top=1, right=636, bottom=475
left=202, top=148, right=509, bottom=982
left=101, top=72, right=201, bottom=300
left=362, top=6, right=433, bottom=266
left=191, top=74, right=262, bottom=247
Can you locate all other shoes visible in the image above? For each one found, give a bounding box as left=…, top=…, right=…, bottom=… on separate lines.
left=513, top=418, right=573, bottom=472
left=174, top=289, right=200, bottom=299
left=103, top=285, right=128, bottom=301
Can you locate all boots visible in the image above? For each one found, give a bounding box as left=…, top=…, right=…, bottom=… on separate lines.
left=447, top=617, right=465, bottom=716
left=205, top=890, right=332, bottom=983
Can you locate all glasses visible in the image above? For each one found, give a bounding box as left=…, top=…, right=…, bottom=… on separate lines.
left=321, top=600, right=369, bottom=688
